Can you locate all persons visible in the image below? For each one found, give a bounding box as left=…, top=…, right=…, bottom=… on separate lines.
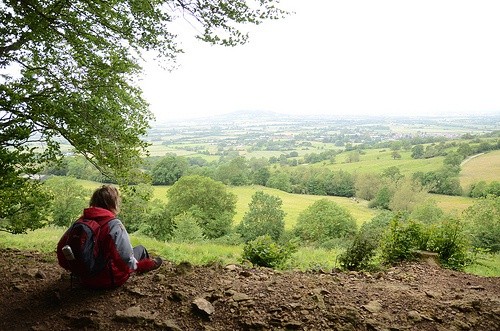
left=57, top=186, right=163, bottom=289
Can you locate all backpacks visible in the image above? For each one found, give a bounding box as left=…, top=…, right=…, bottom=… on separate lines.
left=56, top=216, right=116, bottom=277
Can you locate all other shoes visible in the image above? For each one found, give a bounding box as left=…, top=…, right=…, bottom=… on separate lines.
left=144, top=257, right=162, bottom=274
